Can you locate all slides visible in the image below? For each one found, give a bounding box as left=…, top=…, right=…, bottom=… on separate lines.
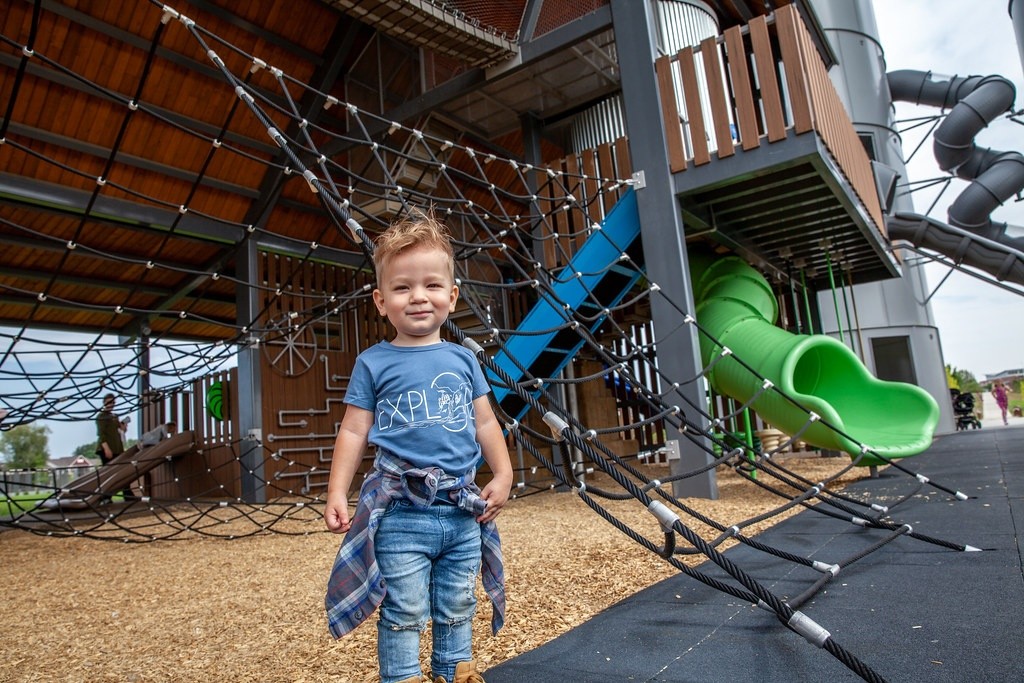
left=887, top=67, right=1022, bottom=287
left=35, top=429, right=194, bottom=511
left=694, top=254, right=942, bottom=467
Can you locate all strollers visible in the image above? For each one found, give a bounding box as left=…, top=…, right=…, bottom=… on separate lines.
left=952, top=391, right=983, bottom=430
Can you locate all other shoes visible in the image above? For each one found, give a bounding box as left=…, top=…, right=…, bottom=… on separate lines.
left=428, top=658, right=486, bottom=683
left=101, top=499, right=114, bottom=505
left=394, top=674, right=432, bottom=683
left=124, top=496, right=141, bottom=501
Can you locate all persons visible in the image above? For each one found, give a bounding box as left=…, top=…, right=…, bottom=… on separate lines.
left=324, top=199, right=513, bottom=683
left=96, top=394, right=142, bottom=506
left=992, top=378, right=1014, bottom=426
